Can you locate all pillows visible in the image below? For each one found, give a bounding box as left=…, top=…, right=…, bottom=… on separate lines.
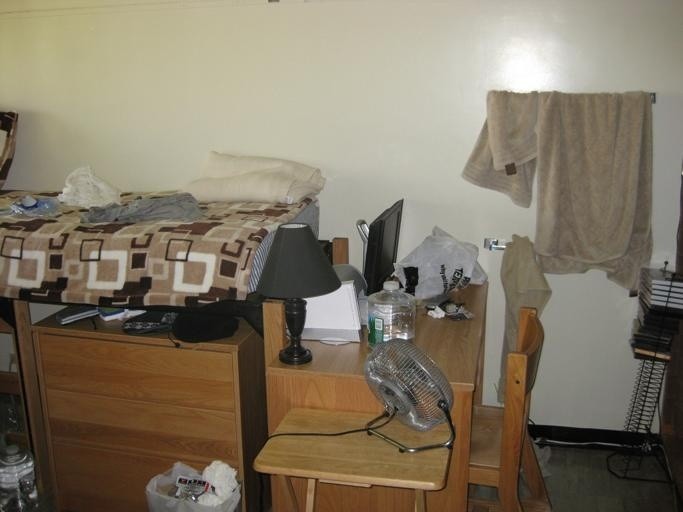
left=0, top=112, right=18, bottom=193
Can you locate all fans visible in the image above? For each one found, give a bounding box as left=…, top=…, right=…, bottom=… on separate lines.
left=364, top=338, right=456, bottom=453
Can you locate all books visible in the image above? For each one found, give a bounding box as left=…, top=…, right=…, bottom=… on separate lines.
left=629, top=268, right=683, bottom=353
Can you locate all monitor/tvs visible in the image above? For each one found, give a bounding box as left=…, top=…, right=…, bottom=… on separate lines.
left=364, top=199, right=403, bottom=297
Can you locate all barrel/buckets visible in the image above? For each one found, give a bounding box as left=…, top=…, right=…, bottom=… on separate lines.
left=0, top=444, right=40, bottom=511
left=367, top=281, right=416, bottom=351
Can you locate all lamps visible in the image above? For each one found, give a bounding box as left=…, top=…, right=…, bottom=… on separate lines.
left=256, top=223, right=341, bottom=365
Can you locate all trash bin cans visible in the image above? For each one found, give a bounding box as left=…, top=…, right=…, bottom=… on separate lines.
left=146, top=464, right=241, bottom=512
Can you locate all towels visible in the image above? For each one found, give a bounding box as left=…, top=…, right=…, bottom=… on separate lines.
left=496, top=233, right=554, bottom=403
left=462, top=89, right=654, bottom=299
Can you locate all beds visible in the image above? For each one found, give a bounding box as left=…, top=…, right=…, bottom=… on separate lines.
left=0, top=190, right=332, bottom=512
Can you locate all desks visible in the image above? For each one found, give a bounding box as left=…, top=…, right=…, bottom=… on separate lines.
left=252, top=408, right=455, bottom=512
left=262, top=238, right=488, bottom=512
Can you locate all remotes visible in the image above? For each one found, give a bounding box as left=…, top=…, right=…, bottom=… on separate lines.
left=425, top=295, right=450, bottom=310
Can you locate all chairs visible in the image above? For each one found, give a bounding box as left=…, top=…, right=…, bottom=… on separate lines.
left=467, top=307, right=552, bottom=512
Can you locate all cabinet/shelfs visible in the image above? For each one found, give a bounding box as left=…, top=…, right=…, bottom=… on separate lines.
left=32, top=306, right=268, bottom=512
